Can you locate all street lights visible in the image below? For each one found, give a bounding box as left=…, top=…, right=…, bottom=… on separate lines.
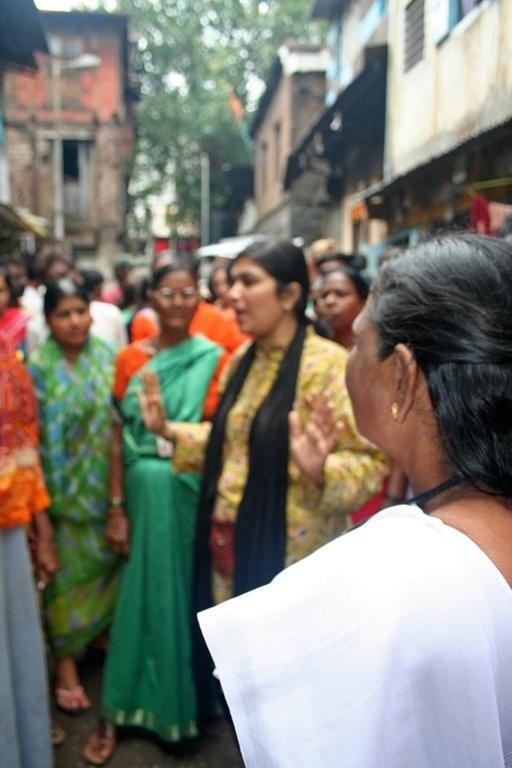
left=51, top=50, right=104, bottom=241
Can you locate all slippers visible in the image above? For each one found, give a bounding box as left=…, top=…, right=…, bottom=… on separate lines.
left=53, top=672, right=90, bottom=716
left=78, top=726, right=118, bottom=767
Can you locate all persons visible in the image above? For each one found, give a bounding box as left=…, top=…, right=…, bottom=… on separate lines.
left=0, top=235, right=414, bottom=768
left=194, top=235, right=512, bottom=768
